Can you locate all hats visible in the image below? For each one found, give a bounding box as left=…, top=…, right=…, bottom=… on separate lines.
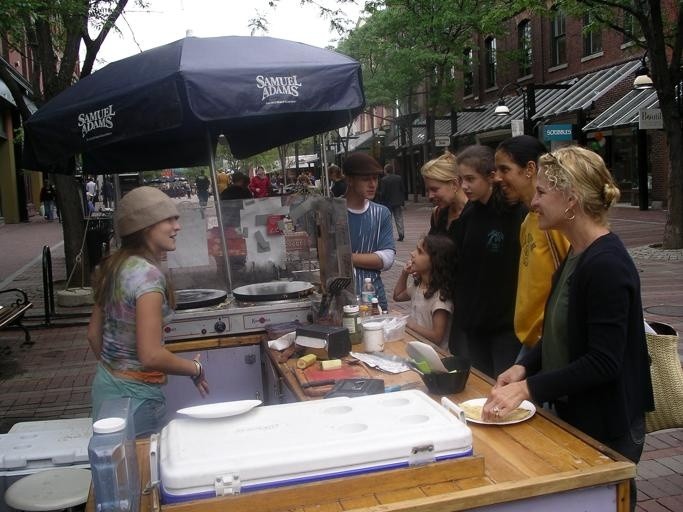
left=111, top=185, right=181, bottom=239
left=342, top=153, right=385, bottom=177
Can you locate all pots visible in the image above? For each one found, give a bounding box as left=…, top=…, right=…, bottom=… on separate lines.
left=178, top=288, right=228, bottom=306
left=235, top=279, right=315, bottom=294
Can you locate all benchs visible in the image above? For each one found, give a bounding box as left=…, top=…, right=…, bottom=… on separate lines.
left=0, top=284, right=35, bottom=349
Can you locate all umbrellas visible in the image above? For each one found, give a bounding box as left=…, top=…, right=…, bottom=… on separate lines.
left=22, top=36, right=366, bottom=293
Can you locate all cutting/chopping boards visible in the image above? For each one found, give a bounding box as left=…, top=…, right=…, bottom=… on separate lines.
left=292, top=365, right=371, bottom=396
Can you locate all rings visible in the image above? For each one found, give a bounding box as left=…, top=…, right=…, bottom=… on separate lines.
left=493, top=407, right=500, bottom=413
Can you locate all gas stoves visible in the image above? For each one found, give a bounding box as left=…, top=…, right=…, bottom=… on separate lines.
left=168, top=297, right=318, bottom=343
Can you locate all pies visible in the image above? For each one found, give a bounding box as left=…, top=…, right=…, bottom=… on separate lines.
left=460, top=404, right=531, bottom=422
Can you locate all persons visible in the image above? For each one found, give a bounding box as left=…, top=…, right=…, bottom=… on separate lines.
left=52, top=185, right=60, bottom=217
left=85, top=178, right=113, bottom=221
left=87, top=186, right=211, bottom=437
left=195, top=163, right=408, bottom=238
left=492, top=135, right=572, bottom=364
left=40, top=178, right=55, bottom=220
left=418, top=154, right=471, bottom=240
left=458, top=146, right=529, bottom=380
left=482, top=146, right=656, bottom=512
left=337, top=152, right=398, bottom=312
left=392, top=234, right=457, bottom=350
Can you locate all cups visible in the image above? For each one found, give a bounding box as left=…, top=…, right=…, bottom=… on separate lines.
left=310, top=297, right=341, bottom=325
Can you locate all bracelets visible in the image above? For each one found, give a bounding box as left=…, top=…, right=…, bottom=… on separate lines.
left=190, top=359, right=203, bottom=384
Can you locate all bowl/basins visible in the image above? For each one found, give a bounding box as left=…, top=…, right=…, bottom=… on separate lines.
left=361, top=315, right=405, bottom=342
left=416, top=357, right=472, bottom=395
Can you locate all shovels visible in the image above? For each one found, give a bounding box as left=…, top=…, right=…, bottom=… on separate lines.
left=321, top=278, right=351, bottom=317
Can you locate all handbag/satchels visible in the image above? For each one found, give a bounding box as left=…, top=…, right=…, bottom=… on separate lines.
left=635, top=318, right=683, bottom=435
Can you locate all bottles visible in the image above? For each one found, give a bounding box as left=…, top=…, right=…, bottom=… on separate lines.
left=359, top=277, right=382, bottom=317
left=341, top=304, right=362, bottom=345
left=362, top=322, right=385, bottom=352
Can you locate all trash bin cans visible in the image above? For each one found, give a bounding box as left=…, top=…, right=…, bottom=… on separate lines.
left=82, top=217, right=112, bottom=272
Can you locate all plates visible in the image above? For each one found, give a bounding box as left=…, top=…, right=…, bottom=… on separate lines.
left=176, top=400, right=263, bottom=419
left=459, top=397, right=536, bottom=426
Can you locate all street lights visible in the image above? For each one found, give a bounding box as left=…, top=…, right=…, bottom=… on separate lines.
left=493, top=81, right=536, bottom=137
left=629, top=41, right=681, bottom=116
left=373, top=115, right=409, bottom=201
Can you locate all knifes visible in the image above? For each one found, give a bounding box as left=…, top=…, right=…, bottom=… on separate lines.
left=278, top=345, right=294, bottom=363
left=299, top=376, right=381, bottom=388
